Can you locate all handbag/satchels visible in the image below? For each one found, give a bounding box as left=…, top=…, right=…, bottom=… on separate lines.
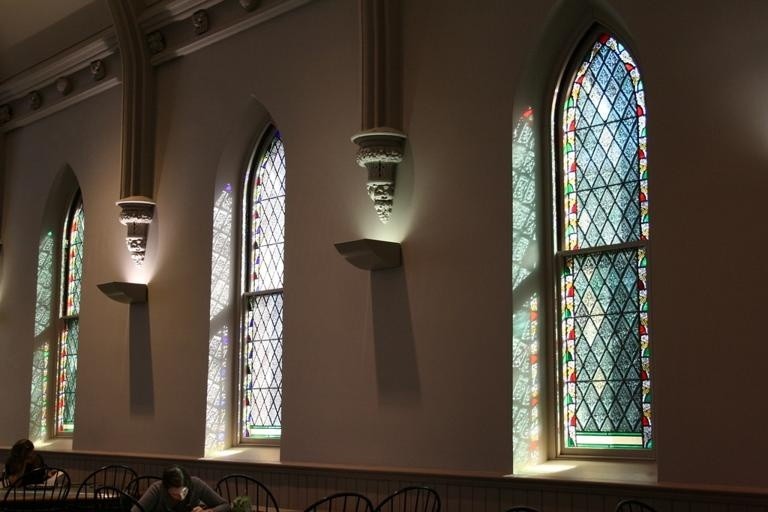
left=22, top=454, right=48, bottom=485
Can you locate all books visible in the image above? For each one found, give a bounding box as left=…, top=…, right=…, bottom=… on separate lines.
left=27, top=470, right=64, bottom=490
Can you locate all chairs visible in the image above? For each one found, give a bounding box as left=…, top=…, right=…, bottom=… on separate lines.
left=303, top=491, right=373, bottom=512
left=372, top=485, right=441, bottom=512
left=0, top=466, right=70, bottom=512
left=120, top=474, right=166, bottom=512
left=93, top=485, right=147, bottom=512
left=214, top=474, right=280, bottom=512
left=74, top=464, right=139, bottom=512
left=614, top=499, right=659, bottom=511
left=500, top=504, right=543, bottom=512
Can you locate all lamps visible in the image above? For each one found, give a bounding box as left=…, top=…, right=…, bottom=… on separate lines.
left=332, top=238, right=403, bottom=271
left=96, top=280, right=149, bottom=304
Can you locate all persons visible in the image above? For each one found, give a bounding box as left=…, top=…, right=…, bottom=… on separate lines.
left=5, top=439, right=58, bottom=487
left=130, top=463, right=230, bottom=511
